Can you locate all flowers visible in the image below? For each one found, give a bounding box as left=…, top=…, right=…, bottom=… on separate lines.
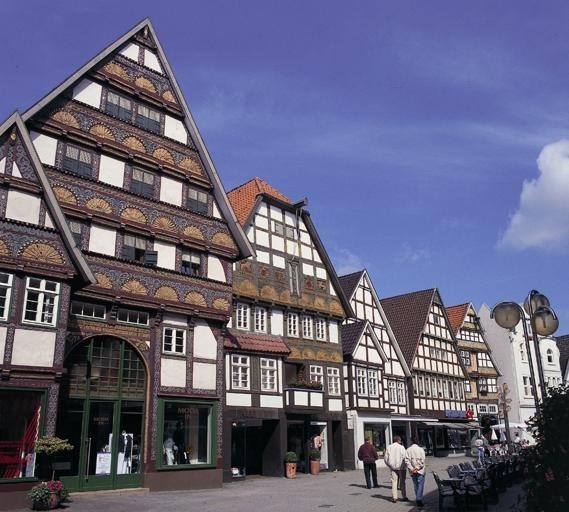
left=26, top=479, right=72, bottom=506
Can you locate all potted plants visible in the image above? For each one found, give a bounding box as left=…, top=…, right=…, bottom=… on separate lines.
left=309, top=449, right=322, bottom=475
left=30, top=436, right=74, bottom=511
left=282, top=451, right=299, bottom=479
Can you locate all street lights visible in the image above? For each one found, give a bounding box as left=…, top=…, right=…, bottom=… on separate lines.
left=488, top=288, right=559, bottom=445
left=496, top=381, right=513, bottom=456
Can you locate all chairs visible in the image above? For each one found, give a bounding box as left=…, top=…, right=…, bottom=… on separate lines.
left=431, top=454, right=531, bottom=512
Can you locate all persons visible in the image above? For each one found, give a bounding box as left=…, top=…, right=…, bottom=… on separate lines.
left=404, top=437, right=427, bottom=507
left=118, top=430, right=132, bottom=474
left=358, top=437, right=380, bottom=488
left=474, top=433, right=531, bottom=458
left=383, top=435, right=408, bottom=503
left=162, top=436, right=188, bottom=465
left=391, top=440, right=406, bottom=489
left=311, top=433, right=323, bottom=457
